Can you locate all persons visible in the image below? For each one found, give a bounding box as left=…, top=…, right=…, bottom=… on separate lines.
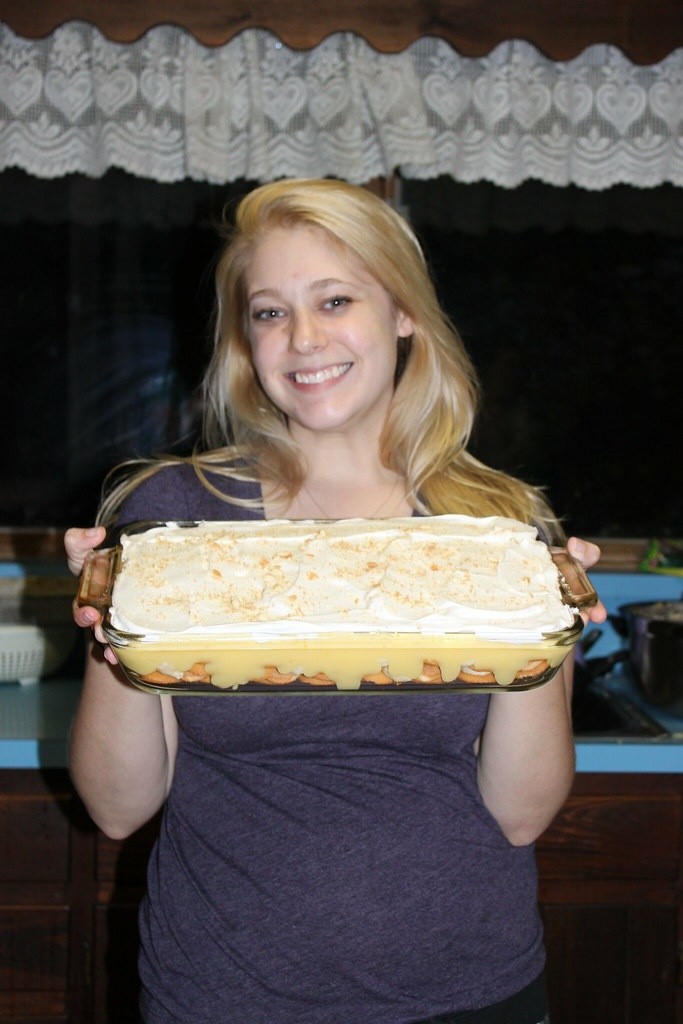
left=64, top=178, right=607, bottom=1024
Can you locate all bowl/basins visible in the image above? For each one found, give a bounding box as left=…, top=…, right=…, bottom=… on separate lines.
left=618, top=597, right=683, bottom=710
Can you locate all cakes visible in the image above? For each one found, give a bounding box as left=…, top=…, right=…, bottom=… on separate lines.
left=108, top=512, right=572, bottom=686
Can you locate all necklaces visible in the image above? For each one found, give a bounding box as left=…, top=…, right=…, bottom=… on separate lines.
left=302, top=474, right=399, bottom=519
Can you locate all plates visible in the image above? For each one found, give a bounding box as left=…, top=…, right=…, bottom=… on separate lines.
left=76, top=517, right=598, bottom=696
